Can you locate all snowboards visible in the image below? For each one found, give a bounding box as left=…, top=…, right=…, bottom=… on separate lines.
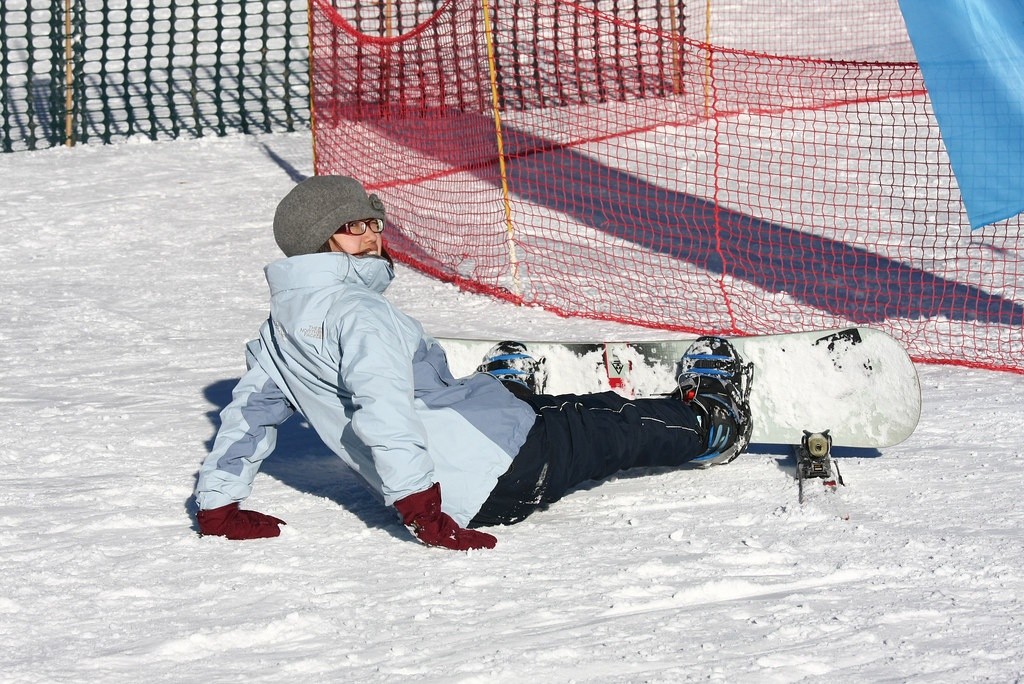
left=429, top=326, right=923, bottom=450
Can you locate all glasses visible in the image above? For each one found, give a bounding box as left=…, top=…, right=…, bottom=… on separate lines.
left=333, top=218, right=384, bottom=235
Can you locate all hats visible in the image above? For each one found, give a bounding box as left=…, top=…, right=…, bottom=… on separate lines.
left=273, top=175, right=385, bottom=258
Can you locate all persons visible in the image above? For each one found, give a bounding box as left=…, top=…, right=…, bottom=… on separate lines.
left=194, top=176, right=754, bottom=548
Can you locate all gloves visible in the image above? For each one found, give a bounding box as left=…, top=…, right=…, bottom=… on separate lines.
left=196, top=502, right=287, bottom=540
left=393, top=481, right=497, bottom=551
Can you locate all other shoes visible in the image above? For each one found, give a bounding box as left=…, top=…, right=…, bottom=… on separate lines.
left=671, top=336, right=741, bottom=462
left=481, top=341, right=534, bottom=392
